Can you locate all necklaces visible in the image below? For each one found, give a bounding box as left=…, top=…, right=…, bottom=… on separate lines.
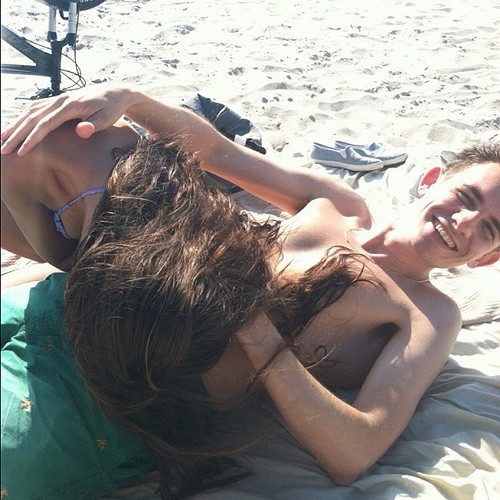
left=346, top=226, right=431, bottom=284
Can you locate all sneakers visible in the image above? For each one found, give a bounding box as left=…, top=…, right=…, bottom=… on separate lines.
left=310, top=142, right=384, bottom=171
left=334, top=140, right=408, bottom=167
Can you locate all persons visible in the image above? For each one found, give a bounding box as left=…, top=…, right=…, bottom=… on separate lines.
left=0, top=112, right=387, bottom=498
left=0, top=81, right=499, bottom=499
